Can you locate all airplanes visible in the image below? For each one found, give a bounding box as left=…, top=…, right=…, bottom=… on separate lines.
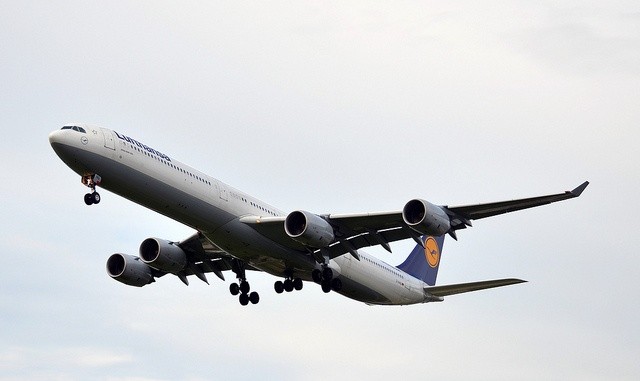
left=48, top=118, right=590, bottom=305
